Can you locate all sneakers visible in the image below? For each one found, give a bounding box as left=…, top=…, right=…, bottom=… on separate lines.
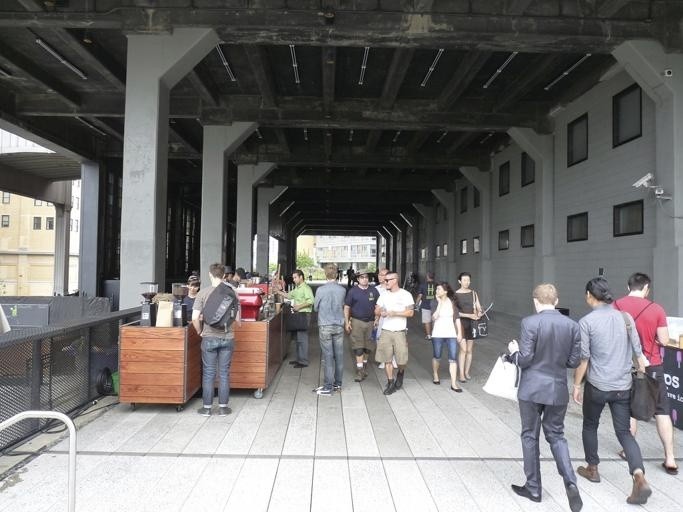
left=317, top=388, right=331, bottom=396
left=198, top=407, right=212, bottom=417
left=384, top=382, right=396, bottom=395
left=425, top=334, right=432, bottom=340
left=459, top=374, right=471, bottom=382
left=395, top=369, right=404, bottom=388
left=332, top=385, right=341, bottom=389
left=354, top=365, right=368, bottom=382
left=218, top=407, right=232, bottom=416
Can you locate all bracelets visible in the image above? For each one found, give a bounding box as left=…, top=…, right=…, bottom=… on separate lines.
left=572, top=383, right=581, bottom=389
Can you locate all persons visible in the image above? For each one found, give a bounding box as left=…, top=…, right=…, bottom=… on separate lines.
left=223, top=264, right=482, bottom=395
left=508, top=284, right=583, bottom=512
left=192, top=263, right=236, bottom=416
left=572, top=277, right=652, bottom=505
left=181, top=275, right=200, bottom=323
left=608, top=271, right=679, bottom=475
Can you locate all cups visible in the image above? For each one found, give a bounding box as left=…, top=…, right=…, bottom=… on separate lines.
left=263, top=305, right=269, bottom=318
left=274, top=302, right=282, bottom=314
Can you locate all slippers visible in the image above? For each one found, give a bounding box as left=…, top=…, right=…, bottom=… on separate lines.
left=662, top=461, right=678, bottom=475
left=619, top=450, right=627, bottom=461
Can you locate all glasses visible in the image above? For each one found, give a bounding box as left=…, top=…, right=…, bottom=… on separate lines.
left=384, top=278, right=393, bottom=283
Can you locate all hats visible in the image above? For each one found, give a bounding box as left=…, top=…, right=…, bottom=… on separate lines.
left=350, top=270, right=374, bottom=282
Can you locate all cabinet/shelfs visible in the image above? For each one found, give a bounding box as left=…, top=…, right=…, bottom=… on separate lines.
left=118, top=302, right=294, bottom=412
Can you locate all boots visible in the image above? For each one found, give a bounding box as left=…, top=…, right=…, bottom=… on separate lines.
left=577, top=465, right=600, bottom=482
left=626, top=472, right=652, bottom=505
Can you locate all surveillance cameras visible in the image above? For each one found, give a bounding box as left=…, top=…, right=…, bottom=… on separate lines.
left=631, top=172, right=662, bottom=191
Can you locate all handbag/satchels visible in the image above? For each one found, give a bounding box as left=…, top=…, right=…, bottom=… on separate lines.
left=482, top=354, right=521, bottom=402
left=629, top=370, right=661, bottom=423
left=202, top=281, right=238, bottom=331
left=286, top=312, right=308, bottom=331
left=469, top=317, right=488, bottom=339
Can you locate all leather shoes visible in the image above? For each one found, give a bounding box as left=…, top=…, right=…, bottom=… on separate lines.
left=289, top=361, right=297, bottom=364
left=433, top=380, right=440, bottom=385
left=451, top=386, right=462, bottom=392
left=294, top=364, right=308, bottom=368
left=566, top=482, right=583, bottom=512
left=511, top=484, right=541, bottom=502
